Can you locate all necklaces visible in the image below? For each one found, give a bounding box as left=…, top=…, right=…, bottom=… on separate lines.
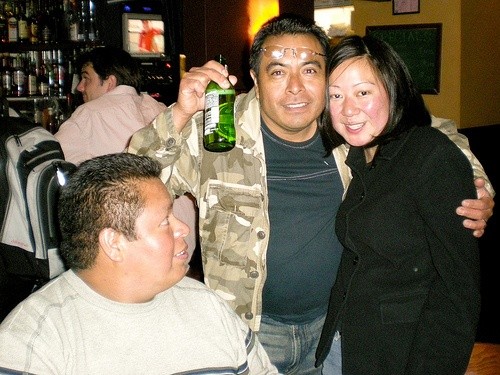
left=0, top=155, right=285, bottom=375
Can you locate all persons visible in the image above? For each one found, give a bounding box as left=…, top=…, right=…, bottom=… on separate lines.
left=53, top=14, right=496, bottom=375
left=138, top=19, right=163, bottom=54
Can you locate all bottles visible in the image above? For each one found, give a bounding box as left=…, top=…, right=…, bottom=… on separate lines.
left=0, top=0, right=95, bottom=136
left=203, top=53, right=236, bottom=153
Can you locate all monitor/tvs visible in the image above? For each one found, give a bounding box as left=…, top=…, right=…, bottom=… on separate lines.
left=122, top=14, right=167, bottom=58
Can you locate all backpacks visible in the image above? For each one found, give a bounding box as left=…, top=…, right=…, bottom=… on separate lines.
left=0, top=104, right=77, bottom=292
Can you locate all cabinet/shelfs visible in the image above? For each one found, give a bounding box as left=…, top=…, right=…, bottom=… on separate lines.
left=0, top=40, right=109, bottom=112
